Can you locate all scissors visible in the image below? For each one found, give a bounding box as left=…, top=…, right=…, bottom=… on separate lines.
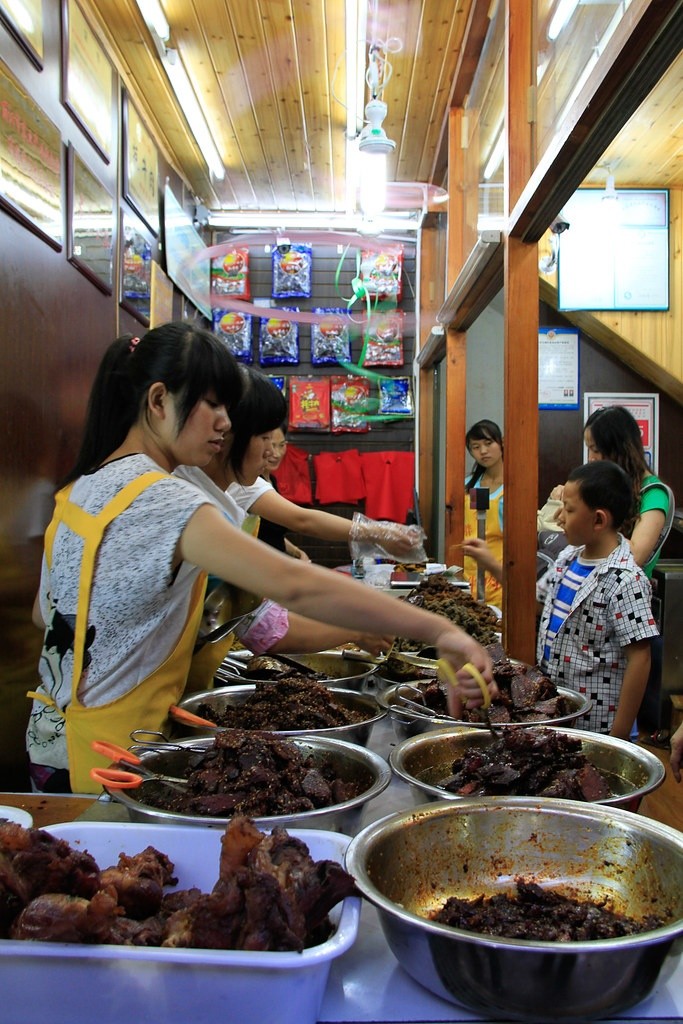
left=434, top=659, right=500, bottom=741
left=90, top=740, right=189, bottom=793
left=127, top=729, right=208, bottom=754
left=387, top=684, right=463, bottom=725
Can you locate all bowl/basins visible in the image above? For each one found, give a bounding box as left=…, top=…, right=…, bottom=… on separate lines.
left=374, top=678, right=592, bottom=734
left=101, top=733, right=391, bottom=830
left=173, top=685, right=388, bottom=750
left=217, top=648, right=379, bottom=692
left=344, top=796, right=683, bottom=1015
left=373, top=656, right=545, bottom=682
left=389, top=725, right=667, bottom=813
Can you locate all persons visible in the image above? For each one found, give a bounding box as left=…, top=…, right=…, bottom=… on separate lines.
left=669, top=721, right=683, bottom=784
left=25, top=321, right=498, bottom=799
left=463, top=459, right=662, bottom=743
left=550, top=406, right=675, bottom=580
left=168, top=362, right=426, bottom=690
left=462, top=419, right=505, bottom=611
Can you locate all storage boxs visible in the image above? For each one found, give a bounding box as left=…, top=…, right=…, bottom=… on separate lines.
left=2, top=821, right=358, bottom=1024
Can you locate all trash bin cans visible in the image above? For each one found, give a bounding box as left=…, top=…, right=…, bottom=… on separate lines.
left=636, top=559, right=683, bottom=730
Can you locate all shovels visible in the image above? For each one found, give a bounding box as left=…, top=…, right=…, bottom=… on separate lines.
left=342, top=646, right=439, bottom=676
left=223, top=656, right=283, bottom=672
left=193, top=581, right=262, bottom=654
